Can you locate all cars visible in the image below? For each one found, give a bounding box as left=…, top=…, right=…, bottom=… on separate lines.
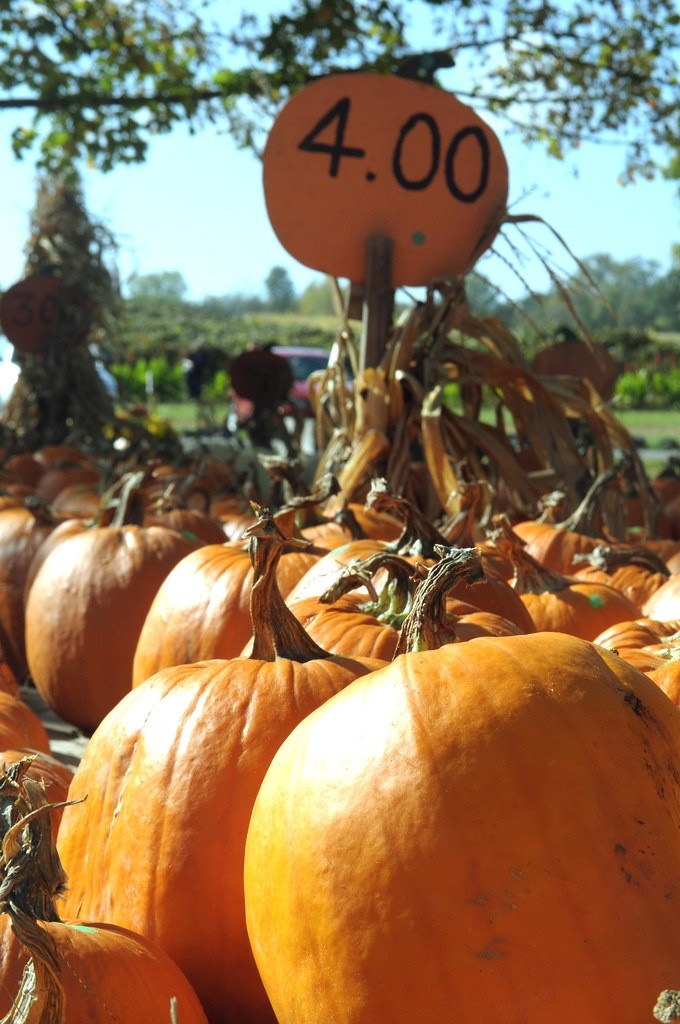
left=232, top=342, right=353, bottom=415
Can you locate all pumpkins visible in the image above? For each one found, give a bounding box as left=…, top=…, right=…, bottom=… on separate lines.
left=0, top=446, right=680, bottom=1024
